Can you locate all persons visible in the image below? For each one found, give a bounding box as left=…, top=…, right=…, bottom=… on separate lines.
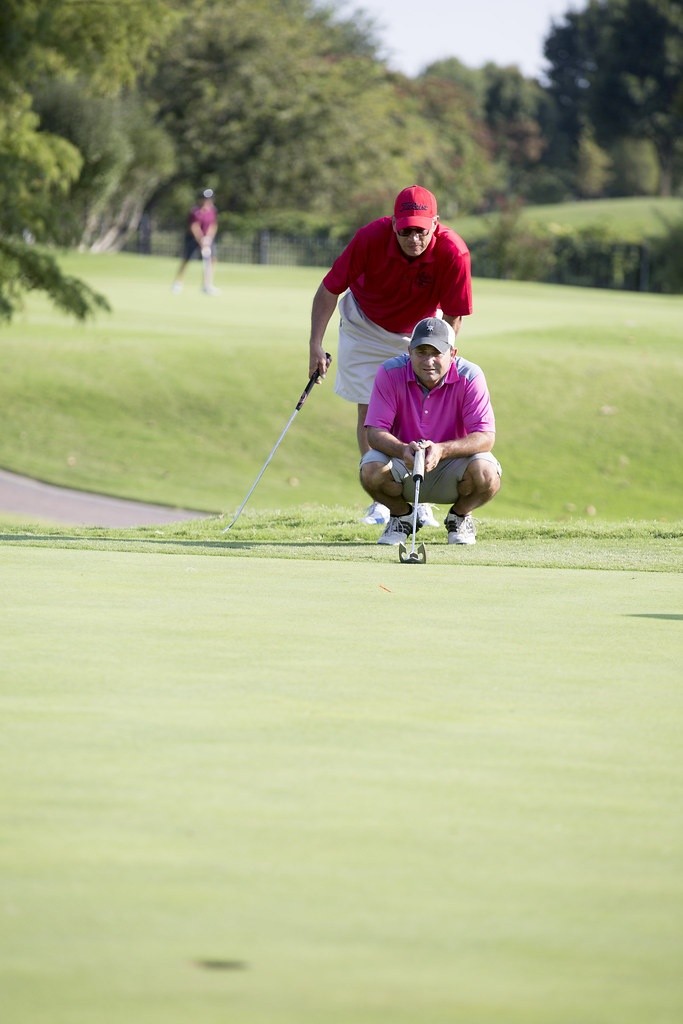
left=309, top=184, right=473, bottom=526
left=359, top=317, right=502, bottom=544
left=172, top=191, right=218, bottom=292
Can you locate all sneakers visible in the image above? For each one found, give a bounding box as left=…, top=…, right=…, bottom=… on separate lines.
left=412, top=502, right=438, bottom=526
left=360, top=501, right=391, bottom=525
left=444, top=507, right=480, bottom=545
left=377, top=503, right=423, bottom=545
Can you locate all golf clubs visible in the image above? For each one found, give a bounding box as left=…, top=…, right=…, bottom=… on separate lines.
left=398, top=438, right=428, bottom=564
left=202, top=247, right=212, bottom=293
left=222, top=351, right=332, bottom=535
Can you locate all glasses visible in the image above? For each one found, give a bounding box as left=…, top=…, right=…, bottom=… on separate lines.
left=397, top=227, right=431, bottom=236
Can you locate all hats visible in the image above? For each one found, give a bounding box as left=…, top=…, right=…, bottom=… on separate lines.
left=409, top=316, right=455, bottom=353
left=395, top=185, right=437, bottom=230
